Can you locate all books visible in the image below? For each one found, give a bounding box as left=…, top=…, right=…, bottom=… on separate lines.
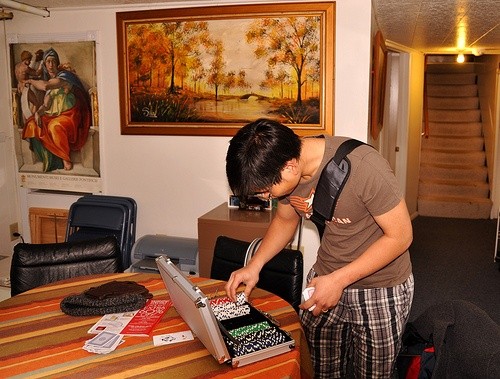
left=88, top=298, right=171, bottom=336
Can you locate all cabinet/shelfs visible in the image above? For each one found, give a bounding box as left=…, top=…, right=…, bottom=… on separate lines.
left=198, top=204, right=294, bottom=281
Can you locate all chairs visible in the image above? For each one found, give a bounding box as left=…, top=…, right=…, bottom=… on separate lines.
left=10, top=196, right=137, bottom=298
left=211, top=236, right=305, bottom=315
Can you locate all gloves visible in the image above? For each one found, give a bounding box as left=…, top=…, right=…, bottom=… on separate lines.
left=84, top=281, right=153, bottom=301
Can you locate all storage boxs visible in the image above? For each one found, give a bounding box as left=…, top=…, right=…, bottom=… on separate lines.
left=155, top=255, right=296, bottom=368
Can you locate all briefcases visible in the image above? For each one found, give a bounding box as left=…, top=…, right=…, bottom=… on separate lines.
left=155, top=255, right=295, bottom=369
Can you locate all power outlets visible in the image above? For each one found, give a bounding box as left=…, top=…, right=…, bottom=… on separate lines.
left=10, top=223, right=19, bottom=241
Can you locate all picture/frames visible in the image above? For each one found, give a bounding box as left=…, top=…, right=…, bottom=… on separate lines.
left=116, top=1, right=336, bottom=141
left=372, top=31, right=388, bottom=138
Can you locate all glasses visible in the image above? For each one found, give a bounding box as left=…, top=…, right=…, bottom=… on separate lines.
left=247, top=165, right=280, bottom=202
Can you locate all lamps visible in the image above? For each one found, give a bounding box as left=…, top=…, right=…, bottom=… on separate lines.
left=456, top=49, right=465, bottom=63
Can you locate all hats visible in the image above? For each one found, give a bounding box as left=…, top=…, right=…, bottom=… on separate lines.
left=59, top=292, right=146, bottom=316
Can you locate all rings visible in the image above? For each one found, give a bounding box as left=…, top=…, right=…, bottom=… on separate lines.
left=322, top=309, right=328, bottom=313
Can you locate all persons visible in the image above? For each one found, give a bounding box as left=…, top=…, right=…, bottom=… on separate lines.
left=224, top=119, right=417, bottom=379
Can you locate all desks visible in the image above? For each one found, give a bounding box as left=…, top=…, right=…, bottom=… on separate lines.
left=0, top=271, right=304, bottom=379
